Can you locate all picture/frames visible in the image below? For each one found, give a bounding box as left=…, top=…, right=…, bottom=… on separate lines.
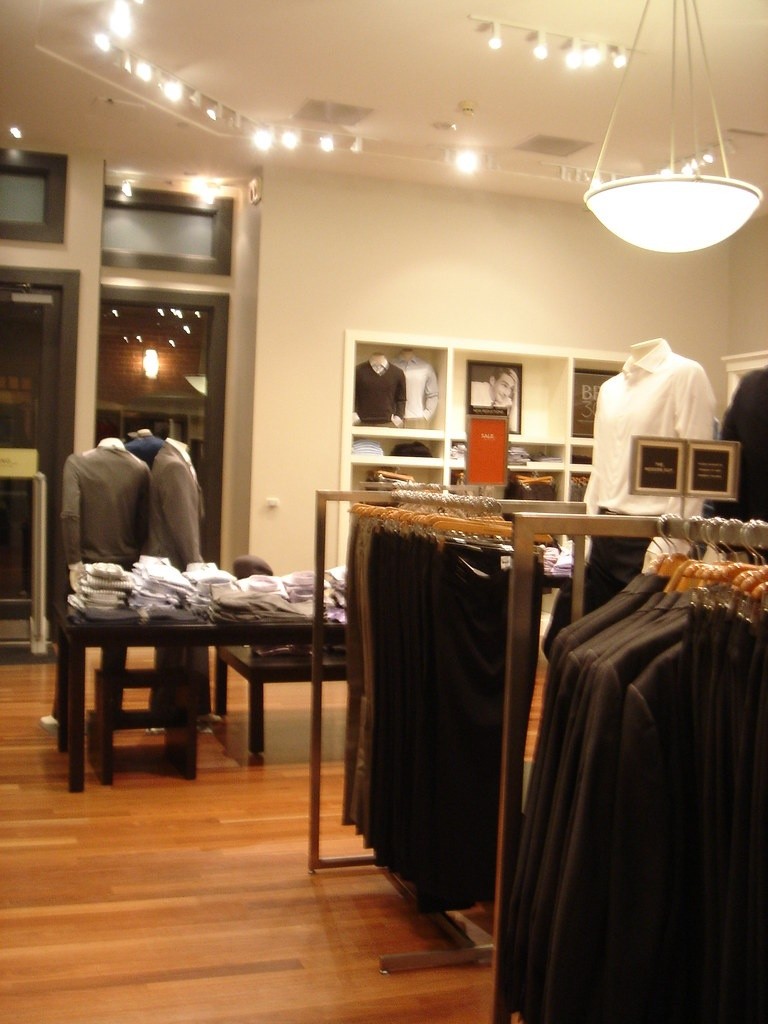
left=465, top=358, right=524, bottom=437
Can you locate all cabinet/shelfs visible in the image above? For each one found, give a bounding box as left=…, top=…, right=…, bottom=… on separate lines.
left=337, top=329, right=629, bottom=569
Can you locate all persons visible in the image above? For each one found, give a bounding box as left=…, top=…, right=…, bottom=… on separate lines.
left=125, top=428, right=164, bottom=470
left=41, top=437, right=150, bottom=726
left=701, top=363, right=768, bottom=567
left=149, top=437, right=222, bottom=724
left=354, top=353, right=406, bottom=428
left=542, top=337, right=716, bottom=664
left=388, top=348, right=439, bottom=429
left=470, top=367, right=519, bottom=433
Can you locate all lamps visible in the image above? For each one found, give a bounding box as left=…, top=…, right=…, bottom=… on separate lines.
left=488, top=22, right=628, bottom=69
left=583, top=0, right=763, bottom=254
left=560, top=167, right=618, bottom=185
left=123, top=52, right=242, bottom=129
left=351, top=136, right=364, bottom=153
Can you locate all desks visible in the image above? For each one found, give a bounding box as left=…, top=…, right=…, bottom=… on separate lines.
left=214, top=642, right=346, bottom=753
left=49, top=601, right=351, bottom=793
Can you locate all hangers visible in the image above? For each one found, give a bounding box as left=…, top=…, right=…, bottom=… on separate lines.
left=347, top=483, right=554, bottom=557
left=643, top=513, right=768, bottom=622
